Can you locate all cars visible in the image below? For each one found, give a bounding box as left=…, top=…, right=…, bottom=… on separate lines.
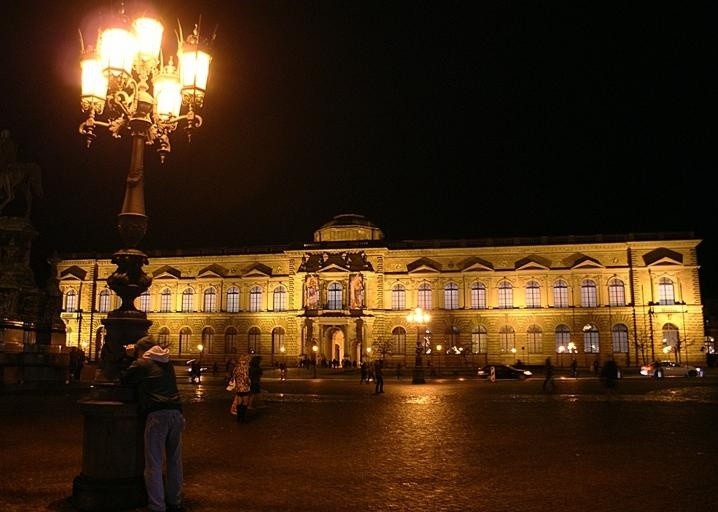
left=478, top=364, right=533, bottom=380
left=641, top=359, right=704, bottom=380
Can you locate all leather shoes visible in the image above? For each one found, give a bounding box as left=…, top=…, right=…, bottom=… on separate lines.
left=136, top=506, right=153, bottom=511
left=164, top=496, right=182, bottom=510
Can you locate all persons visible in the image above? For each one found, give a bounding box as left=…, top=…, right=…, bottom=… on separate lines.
left=298, top=358, right=357, bottom=370
left=540, top=355, right=557, bottom=390
left=246, top=354, right=270, bottom=409
left=191, top=361, right=202, bottom=384
left=358, top=362, right=368, bottom=385
left=229, top=353, right=251, bottom=417
left=114, top=334, right=189, bottom=511
left=212, top=361, right=220, bottom=373
left=375, top=362, right=387, bottom=395
left=568, top=356, right=581, bottom=378
left=367, top=362, right=376, bottom=381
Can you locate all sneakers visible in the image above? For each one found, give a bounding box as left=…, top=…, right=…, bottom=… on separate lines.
left=230, top=406, right=238, bottom=415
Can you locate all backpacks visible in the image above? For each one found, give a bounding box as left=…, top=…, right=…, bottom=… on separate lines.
left=226, top=378, right=237, bottom=393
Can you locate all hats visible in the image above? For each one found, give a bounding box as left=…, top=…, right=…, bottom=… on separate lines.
left=138, top=334, right=155, bottom=351
left=238, top=355, right=249, bottom=361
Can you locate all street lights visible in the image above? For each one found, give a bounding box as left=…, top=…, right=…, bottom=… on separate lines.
left=436, top=344, right=442, bottom=373
left=196, top=344, right=204, bottom=361
left=511, top=348, right=516, bottom=364
left=568, top=342, right=578, bottom=375
left=406, top=308, right=430, bottom=383
left=77, top=0, right=219, bottom=480
left=312, top=345, right=318, bottom=379
left=663, top=340, right=673, bottom=363
left=366, top=348, right=371, bottom=373
left=558, top=346, right=565, bottom=367
